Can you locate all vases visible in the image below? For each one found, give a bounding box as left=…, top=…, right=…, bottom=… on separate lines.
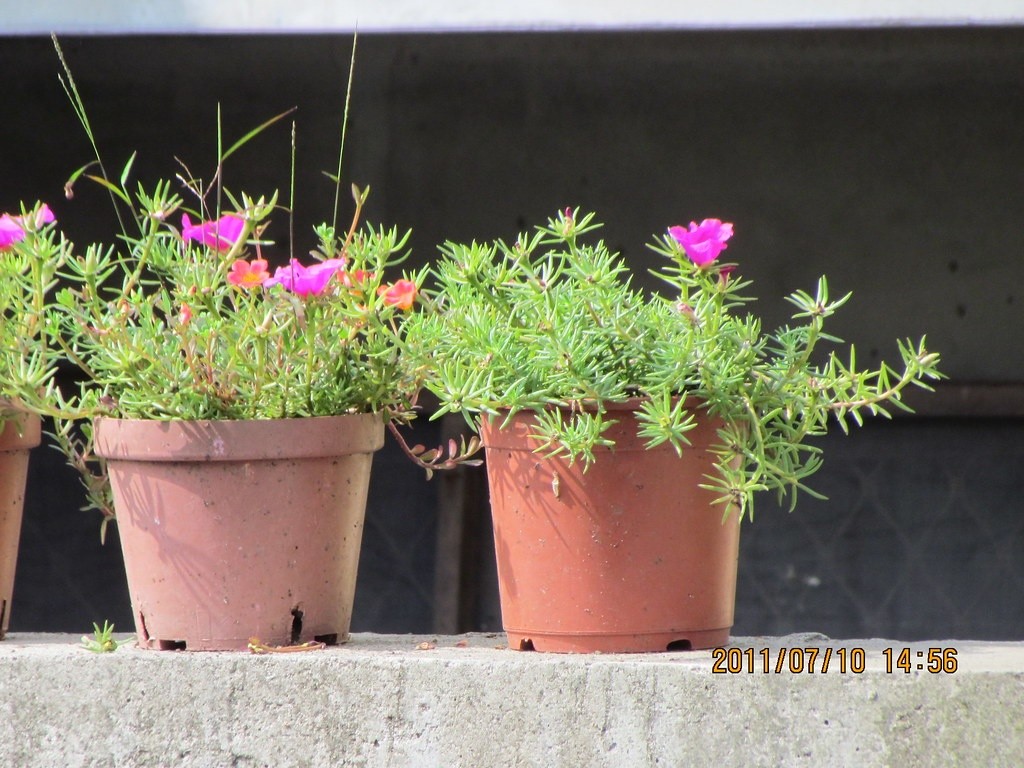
left=478, top=399, right=753, bottom=652
left=92, top=411, right=386, bottom=652
left=1, top=390, right=45, bottom=645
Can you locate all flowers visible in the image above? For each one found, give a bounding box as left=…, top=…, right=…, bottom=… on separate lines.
left=0, top=30, right=953, bottom=515
